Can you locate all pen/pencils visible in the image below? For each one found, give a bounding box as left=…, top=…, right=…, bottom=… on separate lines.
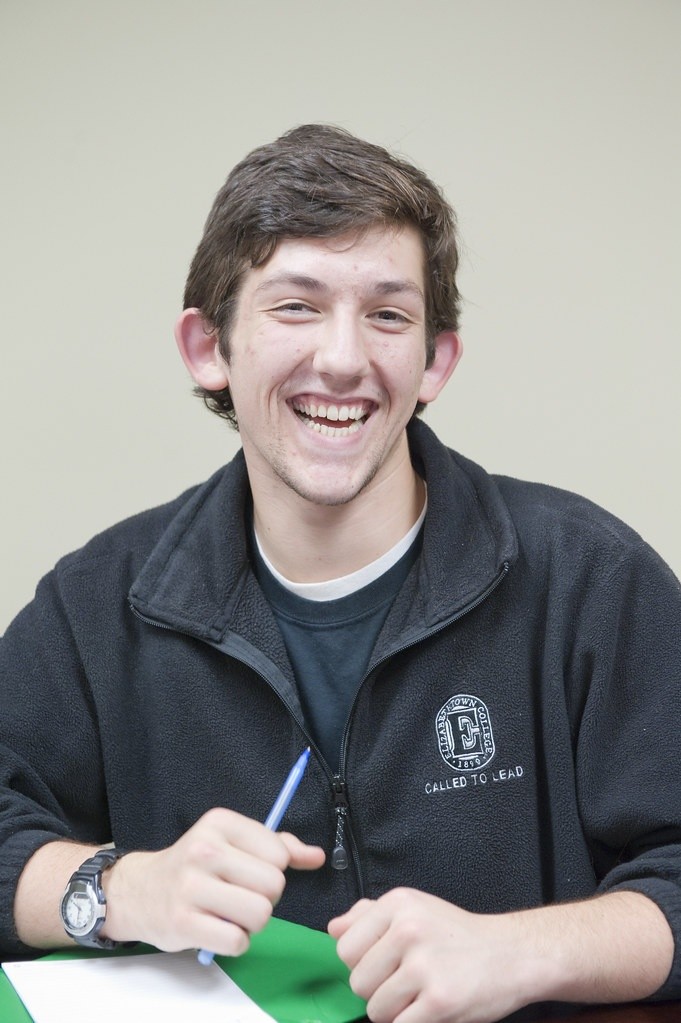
left=192, top=746, right=312, bottom=965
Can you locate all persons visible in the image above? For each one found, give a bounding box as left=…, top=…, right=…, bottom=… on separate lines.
left=1, top=117, right=681, bottom=1023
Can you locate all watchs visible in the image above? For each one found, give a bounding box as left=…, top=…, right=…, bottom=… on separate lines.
left=61, top=847, right=140, bottom=952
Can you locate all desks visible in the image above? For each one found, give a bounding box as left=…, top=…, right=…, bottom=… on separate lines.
left=354, top=995, right=681, bottom=1023
